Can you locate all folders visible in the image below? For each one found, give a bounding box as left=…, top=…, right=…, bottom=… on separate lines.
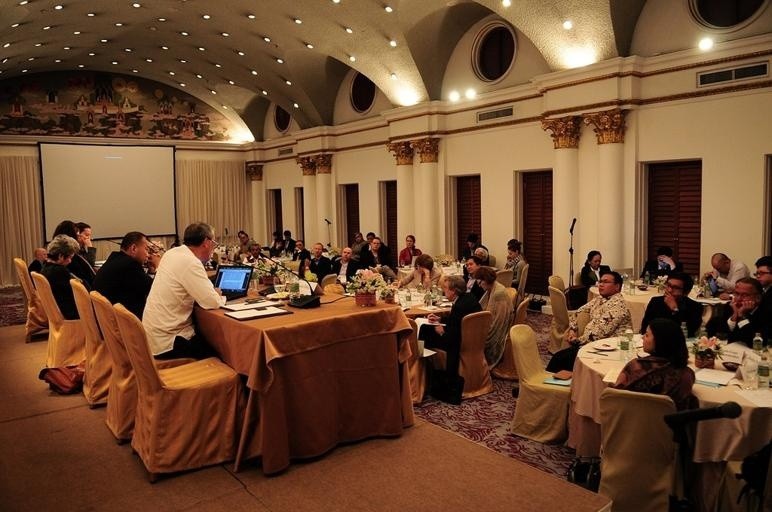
left=224, top=306, right=294, bottom=321
left=220, top=298, right=283, bottom=311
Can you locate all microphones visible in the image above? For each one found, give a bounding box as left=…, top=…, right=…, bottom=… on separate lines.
left=665, top=402, right=741, bottom=426
left=103, top=238, right=120, bottom=245
left=146, top=235, right=165, bottom=251
left=224, top=228, right=227, bottom=234
left=324, top=218, right=331, bottom=224
left=257, top=252, right=320, bottom=308
left=220, top=256, right=282, bottom=296
left=568, top=217, right=577, bottom=232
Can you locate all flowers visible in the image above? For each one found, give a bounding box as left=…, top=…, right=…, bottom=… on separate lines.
left=432, top=254, right=453, bottom=266
left=702, top=270, right=718, bottom=287
left=691, top=335, right=722, bottom=358
left=379, top=284, right=396, bottom=297
left=346, top=268, right=387, bottom=294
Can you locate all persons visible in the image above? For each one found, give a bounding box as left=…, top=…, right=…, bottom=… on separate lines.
left=238, top=231, right=244, bottom=238
left=463, top=256, right=486, bottom=301
left=282, top=230, right=296, bottom=251
left=309, top=243, right=331, bottom=277
left=504, top=242, right=525, bottom=291
left=29, top=248, right=48, bottom=290
left=399, top=235, right=422, bottom=268
left=474, top=248, right=488, bottom=266
left=234, top=234, right=255, bottom=259
left=475, top=267, right=513, bottom=369
left=366, top=236, right=388, bottom=269
left=641, top=246, right=682, bottom=278
left=702, top=253, right=750, bottom=292
left=398, top=254, right=441, bottom=291
left=53, top=221, right=97, bottom=286
left=753, top=256, right=772, bottom=300
left=419, top=275, right=480, bottom=355
left=464, top=234, right=489, bottom=258
left=141, top=222, right=227, bottom=359
left=76, top=222, right=96, bottom=267
left=242, top=244, right=265, bottom=266
left=350, top=233, right=367, bottom=262
left=705, top=277, right=772, bottom=349
left=581, top=251, right=612, bottom=287
left=40, top=234, right=91, bottom=320
left=332, top=247, right=361, bottom=282
left=292, top=240, right=310, bottom=271
left=507, top=239, right=520, bottom=246
left=360, top=232, right=385, bottom=262
left=91, top=232, right=157, bottom=321
left=615, top=319, right=699, bottom=411
left=511, top=271, right=632, bottom=399
left=641, top=273, right=703, bottom=335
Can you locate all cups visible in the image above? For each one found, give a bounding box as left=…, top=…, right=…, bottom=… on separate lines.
left=400, top=259, right=406, bottom=269
left=247, top=278, right=260, bottom=301
left=274, top=276, right=286, bottom=302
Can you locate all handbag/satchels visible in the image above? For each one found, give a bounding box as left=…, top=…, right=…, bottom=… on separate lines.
left=429, top=369, right=464, bottom=404
left=568, top=456, right=600, bottom=490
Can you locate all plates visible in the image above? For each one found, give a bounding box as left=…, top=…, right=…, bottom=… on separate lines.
left=637, top=351, right=651, bottom=358
left=594, top=342, right=617, bottom=352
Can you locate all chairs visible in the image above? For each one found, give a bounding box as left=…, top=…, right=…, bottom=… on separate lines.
left=599, top=388, right=677, bottom=512
left=31, top=271, right=85, bottom=393
left=433, top=309, right=492, bottom=401
left=14, top=256, right=50, bottom=341
left=91, top=290, right=197, bottom=447
left=495, top=299, right=530, bottom=381
left=548, top=289, right=576, bottom=356
left=113, top=302, right=241, bottom=479
left=549, top=275, right=569, bottom=301
left=321, top=272, right=338, bottom=289
left=519, top=264, right=531, bottom=305
left=70, top=278, right=109, bottom=407
left=499, top=269, right=515, bottom=291
left=510, top=324, right=575, bottom=445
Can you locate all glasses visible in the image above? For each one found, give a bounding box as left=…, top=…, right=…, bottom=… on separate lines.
left=666, top=284, right=684, bottom=290
left=373, top=243, right=381, bottom=245
left=599, top=280, right=618, bottom=284
left=355, top=236, right=360, bottom=239
left=753, top=272, right=772, bottom=276
left=208, top=236, right=219, bottom=248
left=478, top=280, right=482, bottom=287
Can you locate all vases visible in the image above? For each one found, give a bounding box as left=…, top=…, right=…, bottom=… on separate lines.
left=356, top=289, right=377, bottom=307
left=696, top=353, right=715, bottom=368
left=383, top=294, right=396, bottom=306
left=704, top=284, right=714, bottom=298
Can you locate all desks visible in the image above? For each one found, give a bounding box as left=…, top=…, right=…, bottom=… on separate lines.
left=345, top=286, right=454, bottom=402
left=578, top=336, right=772, bottom=503
left=92, top=253, right=415, bottom=475
left=591, top=278, right=737, bottom=325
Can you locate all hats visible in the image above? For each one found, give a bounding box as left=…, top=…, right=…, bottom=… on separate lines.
left=507, top=239, right=522, bottom=246
left=466, top=233, right=480, bottom=243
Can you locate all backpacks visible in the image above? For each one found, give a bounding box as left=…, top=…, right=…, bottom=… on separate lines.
left=735, top=439, right=772, bottom=505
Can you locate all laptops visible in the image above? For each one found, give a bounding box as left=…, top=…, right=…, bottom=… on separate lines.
left=214, top=264, right=252, bottom=299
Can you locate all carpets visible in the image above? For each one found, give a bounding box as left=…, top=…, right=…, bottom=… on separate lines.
left=417, top=311, right=577, bottom=487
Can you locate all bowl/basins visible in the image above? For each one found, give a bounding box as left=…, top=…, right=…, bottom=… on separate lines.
left=722, top=362, right=740, bottom=371
left=637, top=284, right=649, bottom=291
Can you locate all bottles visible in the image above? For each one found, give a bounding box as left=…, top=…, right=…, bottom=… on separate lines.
left=681, top=322, right=687, bottom=338
left=427, top=295, right=432, bottom=311
left=753, top=333, right=763, bottom=356
left=630, top=278, right=635, bottom=295
left=418, top=281, right=423, bottom=293
left=757, top=356, right=769, bottom=390
left=625, top=326, right=633, bottom=350
left=406, top=290, right=411, bottom=307
left=289, top=272, right=300, bottom=302
left=644, top=271, right=650, bottom=286
left=620, top=334, right=629, bottom=363
